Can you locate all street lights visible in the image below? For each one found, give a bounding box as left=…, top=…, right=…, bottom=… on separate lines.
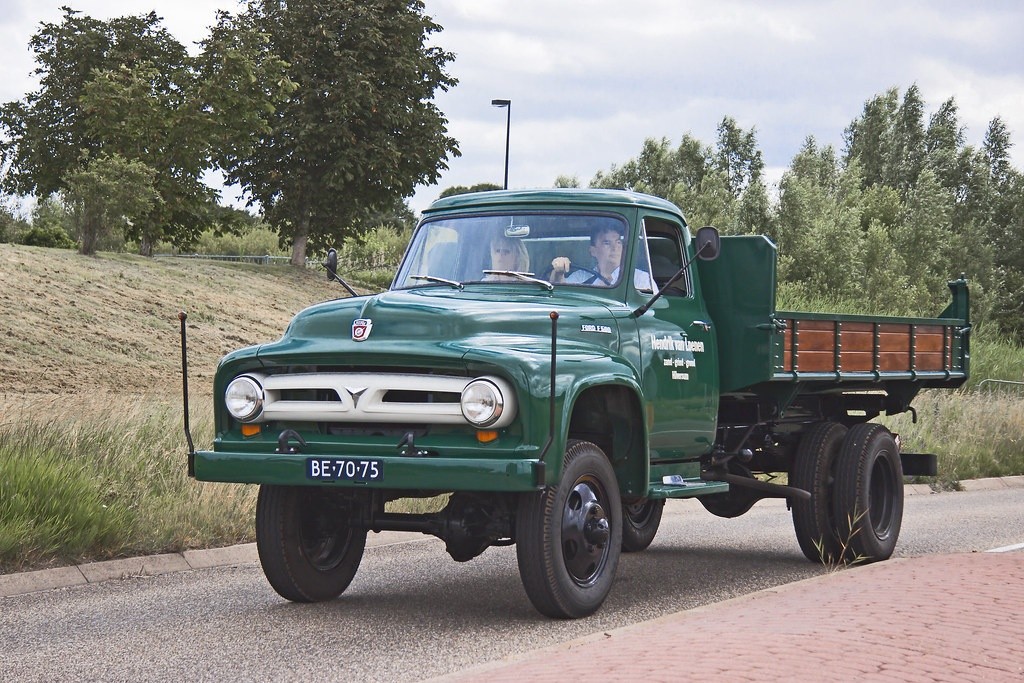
left=492, top=99, right=511, bottom=189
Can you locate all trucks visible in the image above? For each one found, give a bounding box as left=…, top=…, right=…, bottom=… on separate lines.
left=178, top=188, right=973, bottom=620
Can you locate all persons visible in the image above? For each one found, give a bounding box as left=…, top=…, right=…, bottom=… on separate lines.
left=550, top=225, right=659, bottom=293
left=483, top=235, right=529, bottom=281
